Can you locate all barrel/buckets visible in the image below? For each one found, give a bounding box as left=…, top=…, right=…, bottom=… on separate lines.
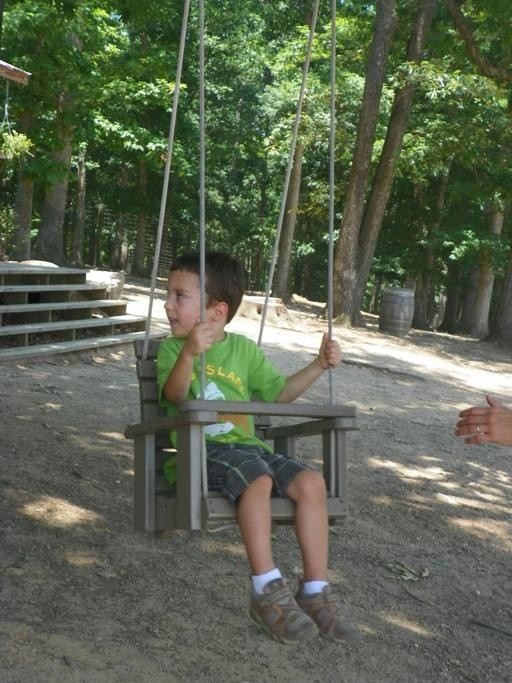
left=379, top=287, right=415, bottom=336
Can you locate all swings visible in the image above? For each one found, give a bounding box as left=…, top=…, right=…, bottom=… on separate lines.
left=125, top=0, right=359, bottom=531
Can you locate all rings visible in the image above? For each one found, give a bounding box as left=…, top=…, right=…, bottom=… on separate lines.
left=476, top=424, right=480, bottom=432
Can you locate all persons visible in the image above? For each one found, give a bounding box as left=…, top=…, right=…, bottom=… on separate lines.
left=156, top=248, right=363, bottom=645
left=454, top=393, right=512, bottom=447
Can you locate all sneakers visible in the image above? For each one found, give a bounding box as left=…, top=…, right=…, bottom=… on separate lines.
left=249, top=577, right=361, bottom=645
